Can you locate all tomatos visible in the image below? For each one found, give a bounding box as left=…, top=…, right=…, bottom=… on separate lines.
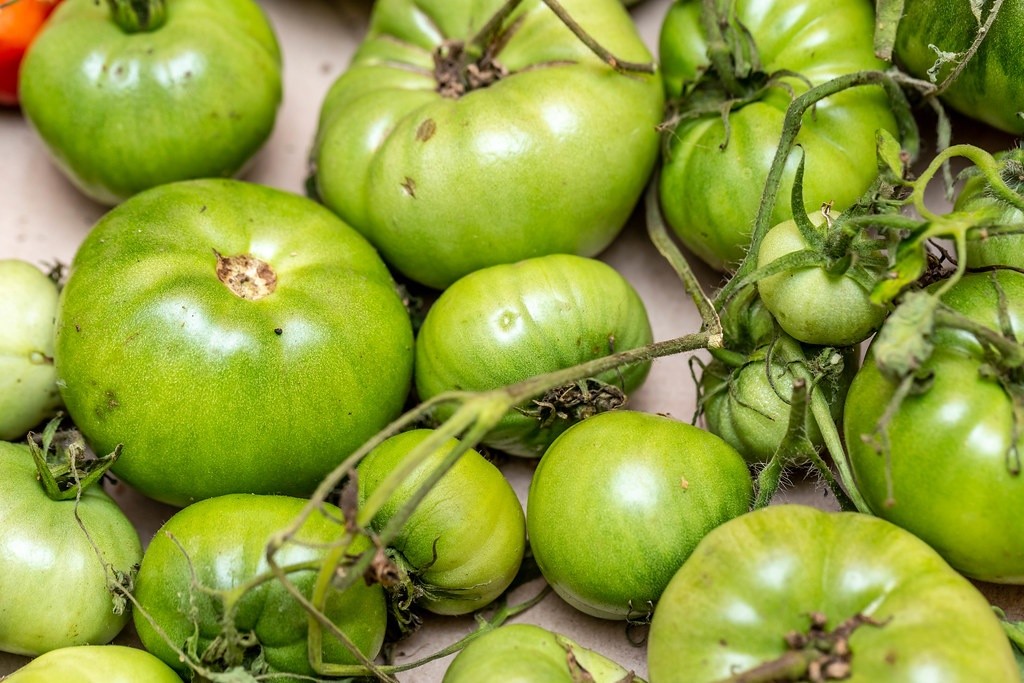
left=0, top=0, right=1024, bottom=683
